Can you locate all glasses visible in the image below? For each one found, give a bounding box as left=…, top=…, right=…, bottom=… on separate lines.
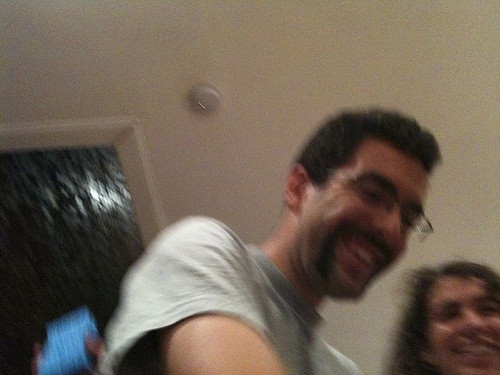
left=329, top=173, right=435, bottom=244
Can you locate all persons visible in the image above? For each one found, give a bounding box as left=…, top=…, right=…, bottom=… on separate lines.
left=383, top=262, right=500, bottom=375
left=93, top=106, right=441, bottom=375
left=29, top=335, right=107, bottom=375
